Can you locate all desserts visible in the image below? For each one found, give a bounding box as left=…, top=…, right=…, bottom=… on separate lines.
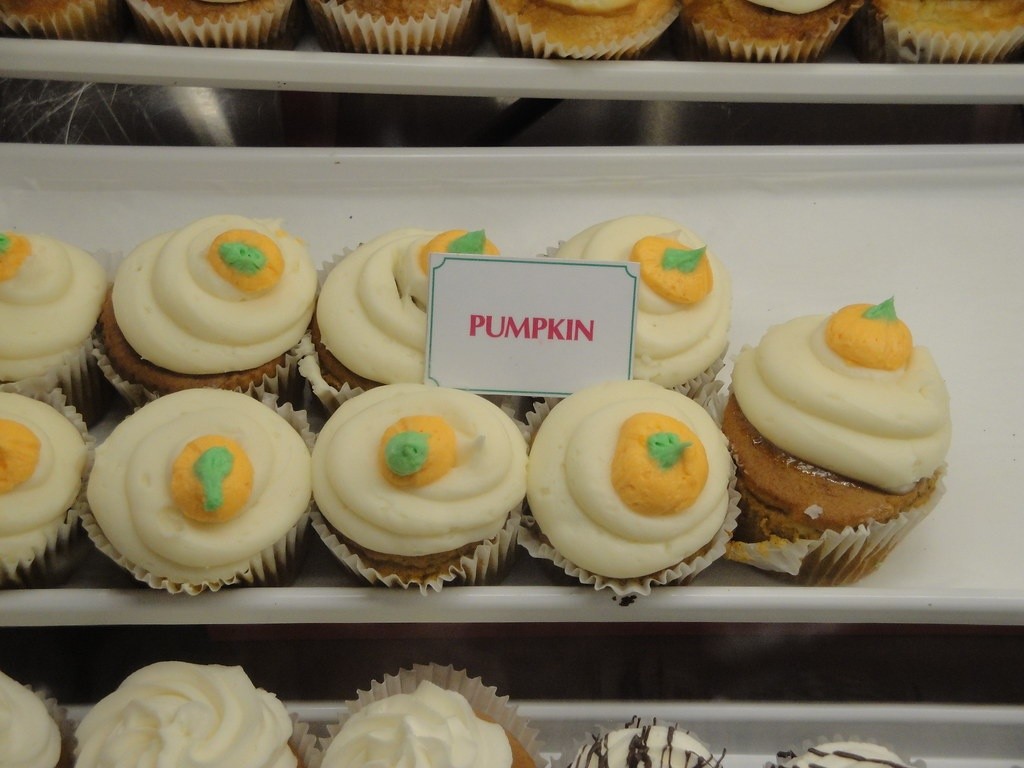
left=1, top=0, right=1024, bottom=768
